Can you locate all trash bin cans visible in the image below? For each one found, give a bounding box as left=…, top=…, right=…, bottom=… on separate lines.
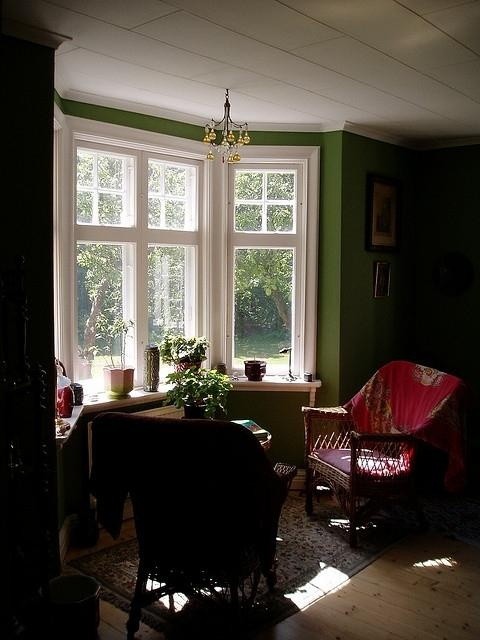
left=38, top=573, right=100, bottom=640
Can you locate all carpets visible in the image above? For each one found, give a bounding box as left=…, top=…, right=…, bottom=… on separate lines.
left=337, top=477, right=479, bottom=550
left=65, top=492, right=414, bottom=640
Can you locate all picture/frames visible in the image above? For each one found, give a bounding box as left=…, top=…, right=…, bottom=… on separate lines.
left=365, top=172, right=400, bottom=254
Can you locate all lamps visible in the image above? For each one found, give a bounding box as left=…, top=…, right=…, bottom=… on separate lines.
left=203, top=87, right=250, bottom=165
left=279, top=347, right=298, bottom=382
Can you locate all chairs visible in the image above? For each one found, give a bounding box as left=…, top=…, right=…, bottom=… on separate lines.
left=89, top=411, right=297, bottom=639
left=300, top=359, right=468, bottom=548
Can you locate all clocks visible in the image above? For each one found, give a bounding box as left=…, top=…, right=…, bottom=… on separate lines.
left=374, top=261, right=390, bottom=299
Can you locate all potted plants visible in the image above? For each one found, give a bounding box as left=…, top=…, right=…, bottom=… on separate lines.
left=163, top=370, right=234, bottom=422
left=242, top=355, right=266, bottom=383
left=93, top=310, right=135, bottom=396
left=159, top=334, right=209, bottom=373
